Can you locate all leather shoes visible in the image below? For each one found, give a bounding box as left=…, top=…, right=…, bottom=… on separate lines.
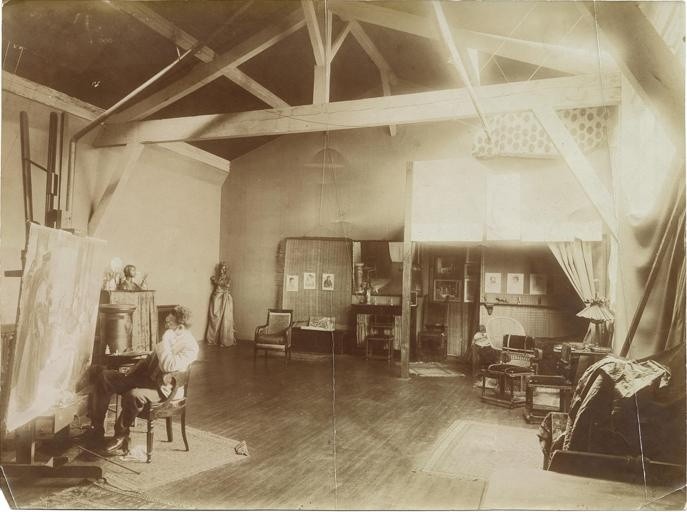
left=103, top=435, right=128, bottom=453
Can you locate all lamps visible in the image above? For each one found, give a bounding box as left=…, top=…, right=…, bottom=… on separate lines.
left=576, top=293, right=613, bottom=351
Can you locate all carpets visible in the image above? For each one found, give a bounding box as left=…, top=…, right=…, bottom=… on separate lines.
left=57, top=417, right=249, bottom=495
left=397, top=361, right=466, bottom=378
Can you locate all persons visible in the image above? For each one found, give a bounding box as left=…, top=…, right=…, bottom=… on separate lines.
left=205, top=263, right=233, bottom=347
left=116, top=264, right=143, bottom=291
left=323, top=274, right=333, bottom=290
left=78, top=304, right=200, bottom=455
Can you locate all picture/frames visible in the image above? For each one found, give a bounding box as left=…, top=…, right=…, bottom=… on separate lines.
left=433, top=279, right=463, bottom=304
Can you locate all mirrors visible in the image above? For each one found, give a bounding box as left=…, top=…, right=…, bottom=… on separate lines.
left=352, top=241, right=402, bottom=297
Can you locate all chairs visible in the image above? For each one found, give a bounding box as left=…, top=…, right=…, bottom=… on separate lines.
left=417, top=294, right=450, bottom=362
left=365, top=314, right=395, bottom=361
left=115, top=350, right=192, bottom=465
left=471, top=325, right=571, bottom=425
left=254, top=308, right=294, bottom=368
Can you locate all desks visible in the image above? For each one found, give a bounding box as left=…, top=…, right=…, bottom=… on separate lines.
left=553, top=343, right=611, bottom=388
left=290, top=326, right=354, bottom=355
